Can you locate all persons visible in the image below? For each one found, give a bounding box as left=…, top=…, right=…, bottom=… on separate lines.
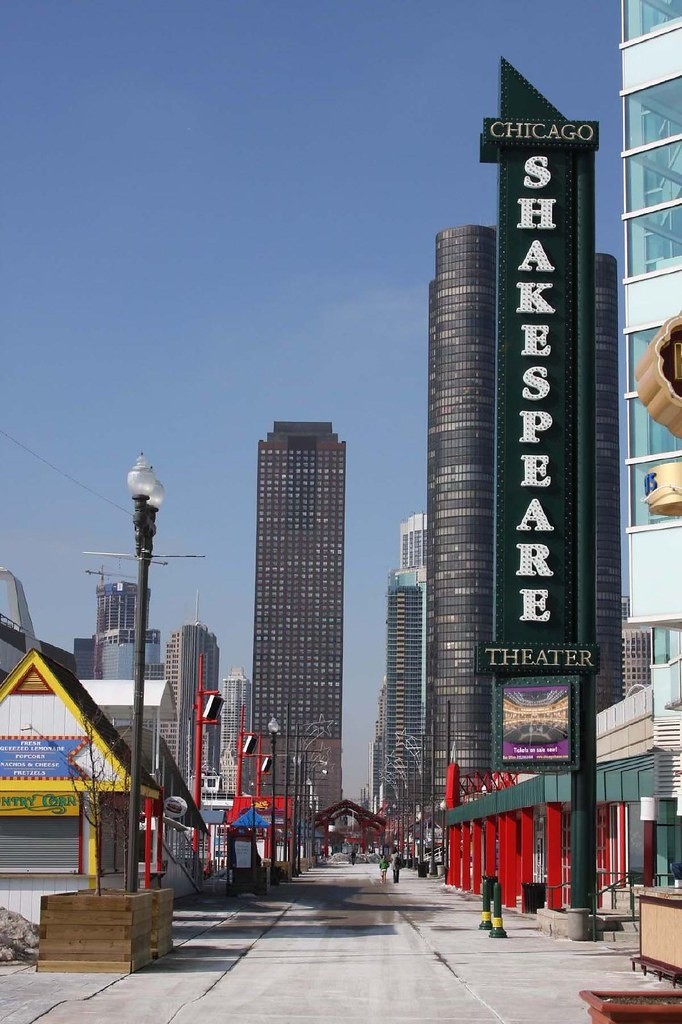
left=379, top=855, right=389, bottom=883
left=390, top=847, right=399, bottom=883
left=351, top=850, right=356, bottom=865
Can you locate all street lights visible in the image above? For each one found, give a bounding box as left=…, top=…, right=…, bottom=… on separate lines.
left=268, top=718, right=281, bottom=886
left=124, top=450, right=168, bottom=894
left=439, top=799, right=446, bottom=866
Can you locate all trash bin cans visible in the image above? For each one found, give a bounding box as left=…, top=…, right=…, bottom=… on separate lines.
left=417, top=864, right=428, bottom=878
left=482, top=875, right=498, bottom=901
left=521, top=881, right=547, bottom=915
